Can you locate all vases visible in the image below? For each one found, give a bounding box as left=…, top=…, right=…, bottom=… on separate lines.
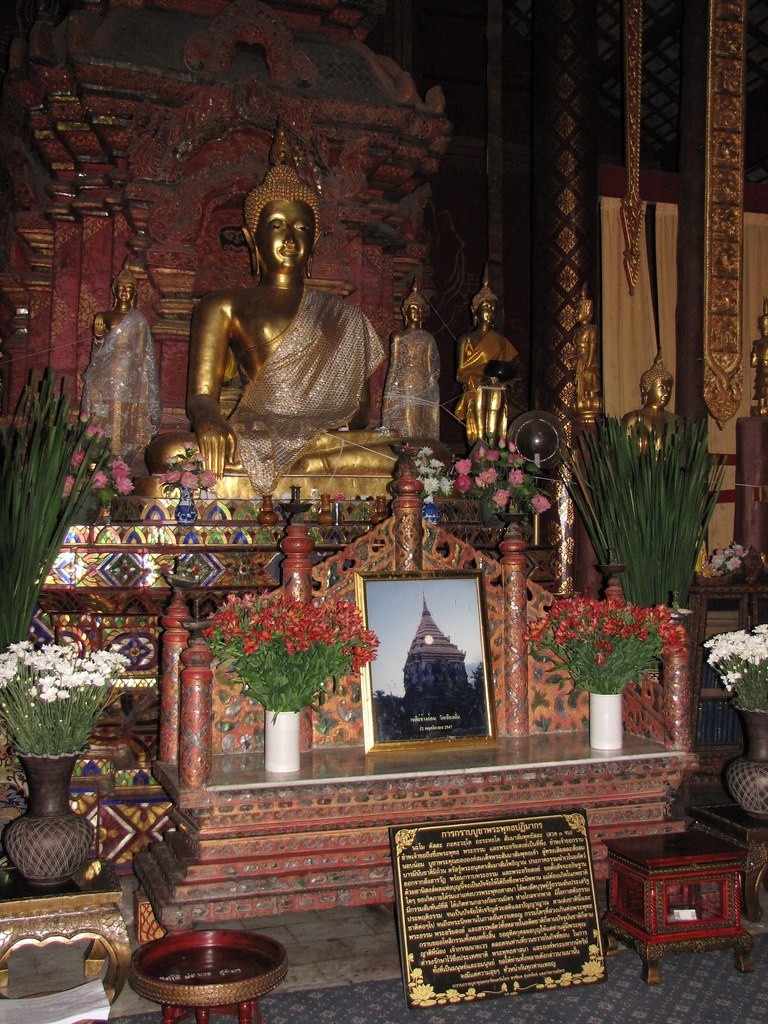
left=589, top=694, right=624, bottom=751
left=6, top=749, right=94, bottom=889
left=726, top=703, right=768, bottom=819
left=265, top=711, right=301, bottom=773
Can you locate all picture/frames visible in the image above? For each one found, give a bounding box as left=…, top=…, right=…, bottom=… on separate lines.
left=353, top=568, right=499, bottom=755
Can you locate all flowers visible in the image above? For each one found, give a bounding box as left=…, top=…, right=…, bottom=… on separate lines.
left=517, top=593, right=685, bottom=710
left=703, top=622, right=768, bottom=712
left=0, top=637, right=127, bottom=758
left=204, top=588, right=383, bottom=717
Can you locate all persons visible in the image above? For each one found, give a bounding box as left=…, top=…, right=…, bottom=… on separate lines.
left=621, top=358, right=683, bottom=463
left=81, top=256, right=161, bottom=464
left=383, top=278, right=440, bottom=439
left=750, top=300, right=768, bottom=406
left=455, top=268, right=522, bottom=447
left=144, top=119, right=452, bottom=474
left=571, top=290, right=599, bottom=399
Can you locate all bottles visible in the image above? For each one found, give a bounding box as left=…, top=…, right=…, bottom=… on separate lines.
left=257, top=496, right=278, bottom=525
left=371, top=496, right=386, bottom=526
left=316, top=495, right=332, bottom=525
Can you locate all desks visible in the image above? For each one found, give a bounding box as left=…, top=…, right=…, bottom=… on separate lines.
left=606, top=832, right=756, bottom=984
left=1, top=858, right=130, bottom=1008
left=687, top=801, right=768, bottom=921
